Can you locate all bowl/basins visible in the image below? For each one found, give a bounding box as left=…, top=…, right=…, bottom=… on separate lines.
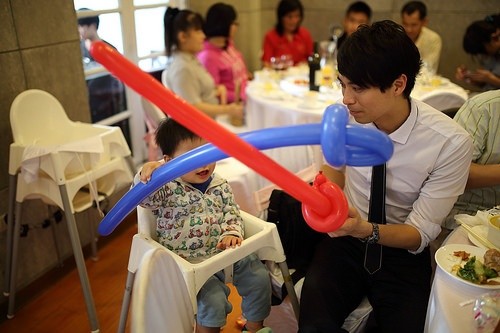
left=486, top=214, right=500, bottom=249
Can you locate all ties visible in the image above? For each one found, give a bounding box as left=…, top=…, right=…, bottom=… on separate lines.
left=364, top=163, right=386, bottom=274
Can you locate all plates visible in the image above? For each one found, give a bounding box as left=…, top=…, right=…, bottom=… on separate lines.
left=434, top=244, right=500, bottom=289
left=468, top=225, right=489, bottom=251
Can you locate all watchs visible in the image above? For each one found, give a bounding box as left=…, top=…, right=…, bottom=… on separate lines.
left=358, top=222, right=380, bottom=246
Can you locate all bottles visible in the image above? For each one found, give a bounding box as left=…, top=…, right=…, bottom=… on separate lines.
left=310, top=34, right=338, bottom=92
left=421, top=61, right=433, bottom=88
left=472, top=290, right=500, bottom=333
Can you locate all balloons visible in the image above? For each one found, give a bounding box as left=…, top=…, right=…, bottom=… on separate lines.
left=97, top=102, right=395, bottom=236
left=89, top=41, right=348, bottom=232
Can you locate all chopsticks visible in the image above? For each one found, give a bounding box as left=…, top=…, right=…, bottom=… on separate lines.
left=458, top=221, right=500, bottom=253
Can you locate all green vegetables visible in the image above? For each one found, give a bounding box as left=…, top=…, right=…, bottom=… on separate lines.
left=457, top=256, right=498, bottom=284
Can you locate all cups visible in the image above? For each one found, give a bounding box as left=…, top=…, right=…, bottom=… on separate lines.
left=304, top=91, right=319, bottom=107
left=271, top=54, right=292, bottom=74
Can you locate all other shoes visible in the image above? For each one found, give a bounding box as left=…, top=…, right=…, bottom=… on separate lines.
left=242, top=322, right=272, bottom=333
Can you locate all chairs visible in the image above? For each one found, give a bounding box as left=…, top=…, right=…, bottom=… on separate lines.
left=421, top=91, right=467, bottom=120
left=4, top=89, right=138, bottom=333
left=140, top=95, right=264, bottom=221
left=115, top=159, right=300, bottom=333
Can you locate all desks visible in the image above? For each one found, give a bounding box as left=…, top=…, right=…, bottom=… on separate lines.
left=424, top=203, right=500, bottom=333
left=244, top=61, right=469, bottom=192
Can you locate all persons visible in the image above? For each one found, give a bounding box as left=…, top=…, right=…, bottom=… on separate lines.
left=430, top=89, right=500, bottom=285
left=129, top=117, right=274, bottom=333
left=400, top=0, right=443, bottom=87
left=295, top=21, right=474, bottom=333
left=261, top=0, right=315, bottom=70
left=455, top=14, right=500, bottom=99
left=330, top=0, right=374, bottom=50
left=74, top=7, right=118, bottom=69
left=161, top=3, right=253, bottom=126
